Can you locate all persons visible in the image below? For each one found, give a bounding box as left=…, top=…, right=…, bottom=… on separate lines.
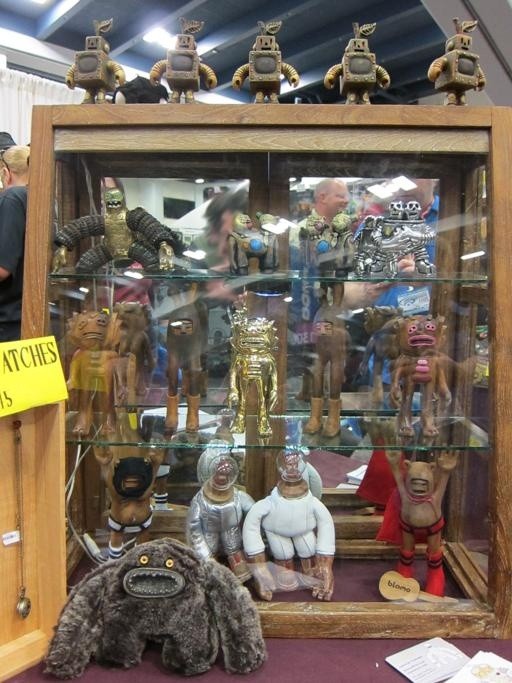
left=0, top=145, right=31, bottom=342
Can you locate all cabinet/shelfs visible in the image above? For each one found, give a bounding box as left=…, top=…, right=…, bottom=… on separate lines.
left=20, top=104, right=512, bottom=640
